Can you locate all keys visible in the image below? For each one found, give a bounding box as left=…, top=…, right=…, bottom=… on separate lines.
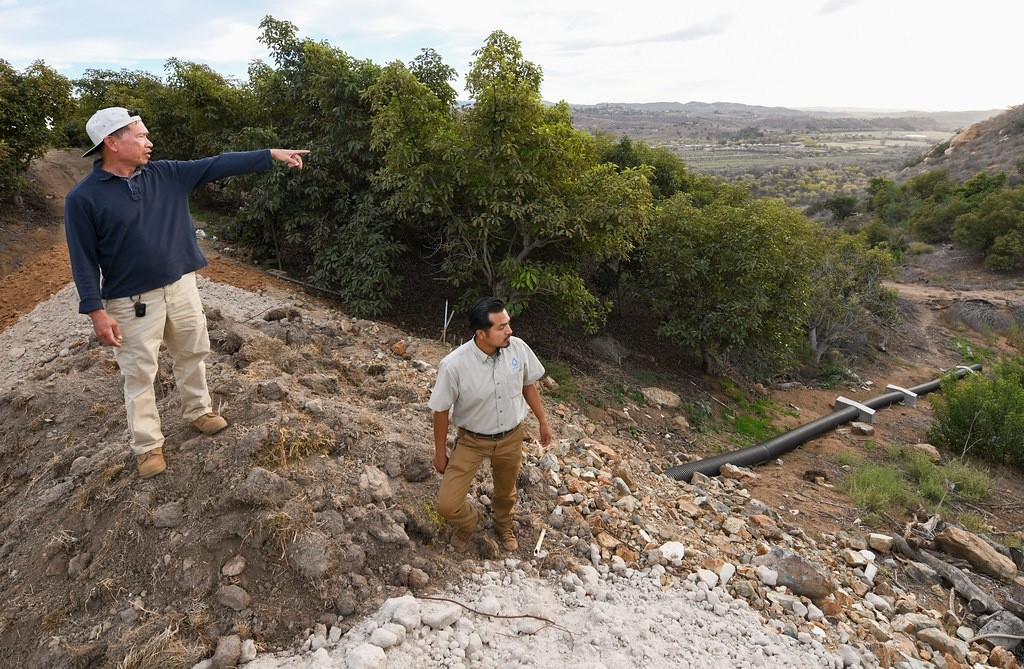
left=135, top=303, right=146, bottom=317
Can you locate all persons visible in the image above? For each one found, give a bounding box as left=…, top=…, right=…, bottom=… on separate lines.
left=426, top=297, right=553, bottom=559
left=65, top=107, right=310, bottom=479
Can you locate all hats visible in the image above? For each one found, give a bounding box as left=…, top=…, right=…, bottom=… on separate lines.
left=82, top=107, right=142, bottom=158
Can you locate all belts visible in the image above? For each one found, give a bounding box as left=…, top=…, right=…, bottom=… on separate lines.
left=459, top=427, right=513, bottom=441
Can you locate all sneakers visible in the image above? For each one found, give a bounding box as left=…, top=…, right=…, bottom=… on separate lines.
left=496, top=528, right=518, bottom=550
left=137, top=447, right=167, bottom=478
left=450, top=510, right=481, bottom=552
left=193, top=413, right=228, bottom=435
